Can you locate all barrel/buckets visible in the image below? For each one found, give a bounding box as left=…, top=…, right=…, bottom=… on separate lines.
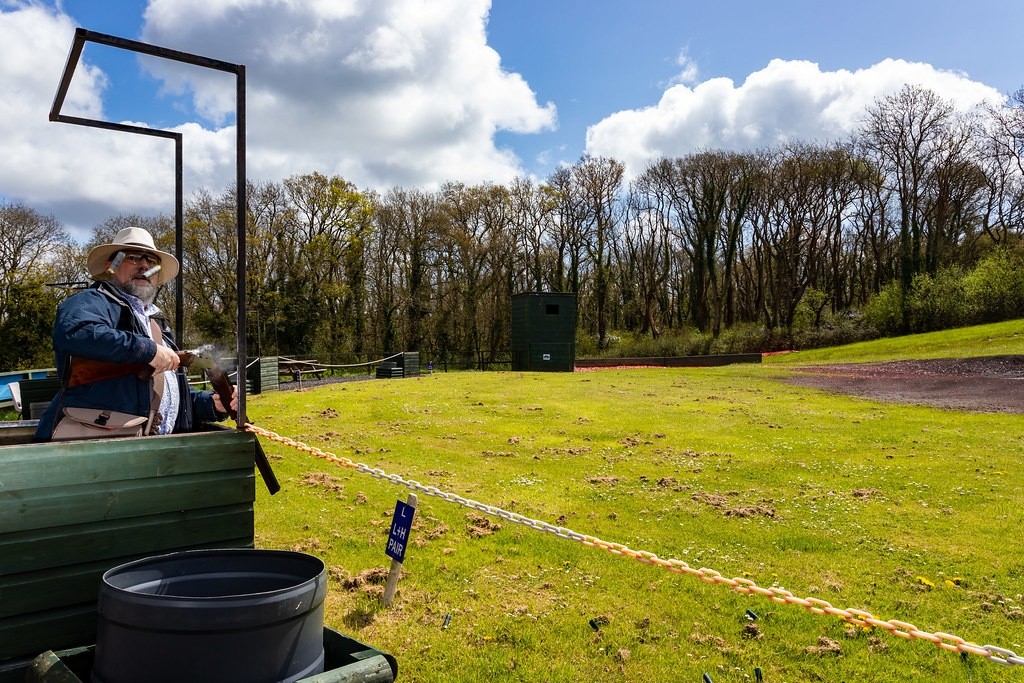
left=91, top=549, right=327, bottom=683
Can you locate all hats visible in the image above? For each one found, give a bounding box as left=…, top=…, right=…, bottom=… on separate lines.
left=87, top=227, right=180, bottom=287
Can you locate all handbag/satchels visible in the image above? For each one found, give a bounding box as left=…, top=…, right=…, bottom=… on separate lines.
left=50, top=406, right=148, bottom=441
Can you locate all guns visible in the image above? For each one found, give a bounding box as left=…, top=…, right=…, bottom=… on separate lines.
left=63, top=345, right=281, bottom=496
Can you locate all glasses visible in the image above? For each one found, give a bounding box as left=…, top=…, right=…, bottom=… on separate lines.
left=124, top=255, right=161, bottom=267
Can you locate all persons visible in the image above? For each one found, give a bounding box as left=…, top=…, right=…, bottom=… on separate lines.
left=32, top=227, right=241, bottom=442
